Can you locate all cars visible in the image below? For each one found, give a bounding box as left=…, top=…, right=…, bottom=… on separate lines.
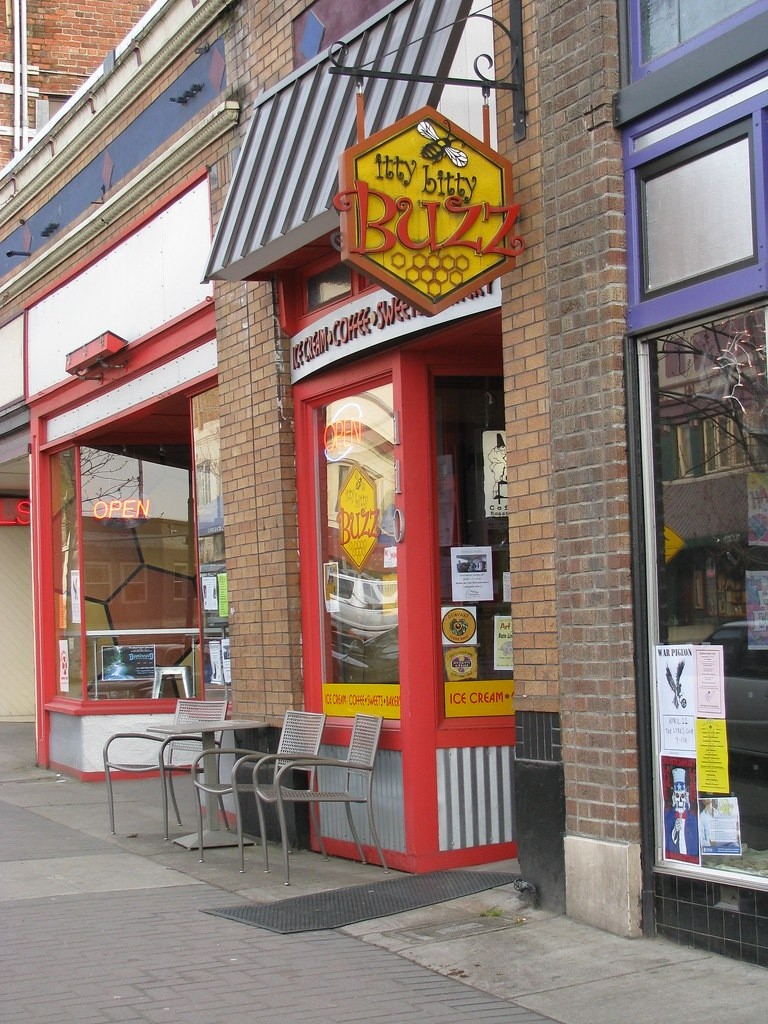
left=330, top=569, right=399, bottom=669
left=78, top=643, right=186, bottom=699
left=695, top=618, right=768, bottom=780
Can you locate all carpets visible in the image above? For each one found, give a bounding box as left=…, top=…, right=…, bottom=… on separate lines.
left=198, top=870, right=523, bottom=935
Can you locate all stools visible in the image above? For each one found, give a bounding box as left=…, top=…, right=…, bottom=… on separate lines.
left=152, top=666, right=193, bottom=698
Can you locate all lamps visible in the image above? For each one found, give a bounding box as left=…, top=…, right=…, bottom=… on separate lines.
left=64, top=330, right=130, bottom=384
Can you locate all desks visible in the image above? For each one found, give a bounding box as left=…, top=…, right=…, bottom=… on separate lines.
left=145, top=719, right=270, bottom=850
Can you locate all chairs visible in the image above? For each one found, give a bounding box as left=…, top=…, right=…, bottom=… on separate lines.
left=100, top=698, right=230, bottom=841
left=190, top=709, right=327, bottom=874
left=251, top=713, right=391, bottom=886
